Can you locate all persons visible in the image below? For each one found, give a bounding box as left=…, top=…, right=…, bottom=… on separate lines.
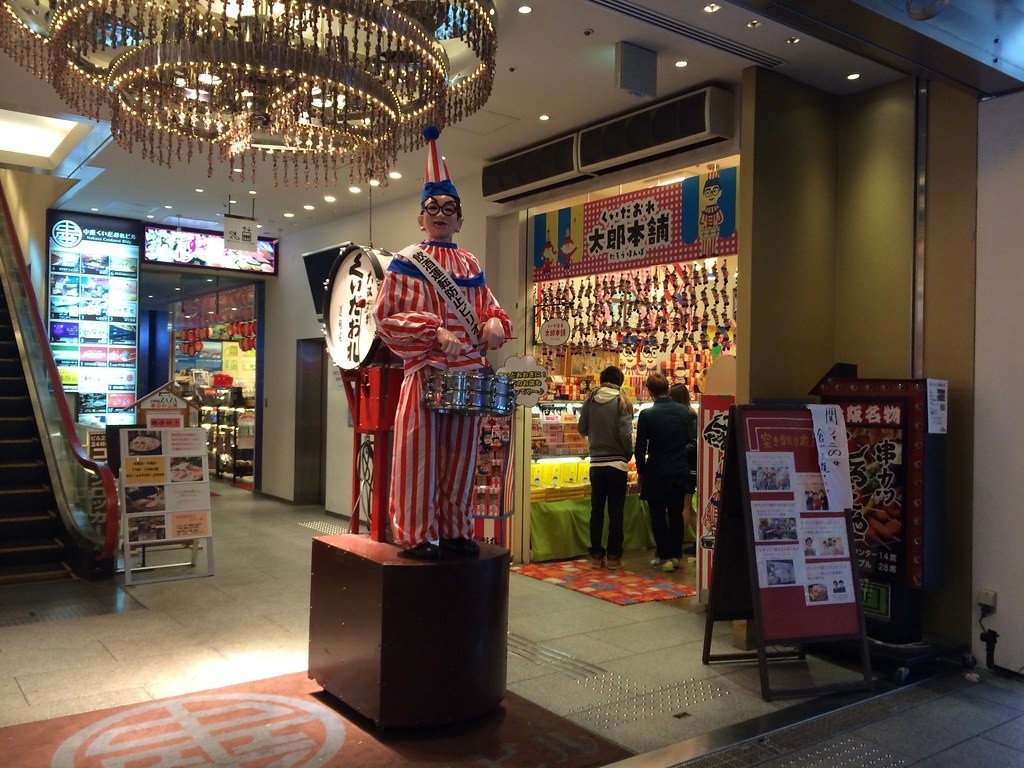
left=577, top=364, right=633, bottom=569
left=805, top=537, right=816, bottom=556
left=833, top=580, right=845, bottom=592
left=754, top=467, right=790, bottom=490
left=372, top=126, right=511, bottom=561
left=540, top=259, right=738, bottom=366
left=806, top=490, right=828, bottom=510
left=635, top=372, right=698, bottom=571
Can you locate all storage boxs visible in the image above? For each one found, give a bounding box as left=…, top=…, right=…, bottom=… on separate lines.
left=623, top=376, right=653, bottom=400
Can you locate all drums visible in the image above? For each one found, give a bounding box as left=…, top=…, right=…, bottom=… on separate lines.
left=422, top=373, right=517, bottom=417
left=319, top=242, right=404, bottom=373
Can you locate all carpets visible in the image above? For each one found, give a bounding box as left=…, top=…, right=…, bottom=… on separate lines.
left=509, top=558, right=696, bottom=606
left=0, top=670, right=640, bottom=768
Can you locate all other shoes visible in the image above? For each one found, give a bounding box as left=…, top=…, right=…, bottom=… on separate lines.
left=606, top=558, right=625, bottom=570
left=671, top=556, right=683, bottom=567
left=650, top=558, right=673, bottom=571
left=591, top=558, right=606, bottom=568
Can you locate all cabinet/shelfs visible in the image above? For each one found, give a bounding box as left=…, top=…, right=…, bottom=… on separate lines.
left=197, top=403, right=256, bottom=484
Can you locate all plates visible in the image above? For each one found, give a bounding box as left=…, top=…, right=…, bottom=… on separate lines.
left=170, top=466, right=202, bottom=482
left=129, top=437, right=161, bottom=452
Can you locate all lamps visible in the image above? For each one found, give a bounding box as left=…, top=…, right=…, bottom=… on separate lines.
left=0, top=0, right=498, bottom=189
